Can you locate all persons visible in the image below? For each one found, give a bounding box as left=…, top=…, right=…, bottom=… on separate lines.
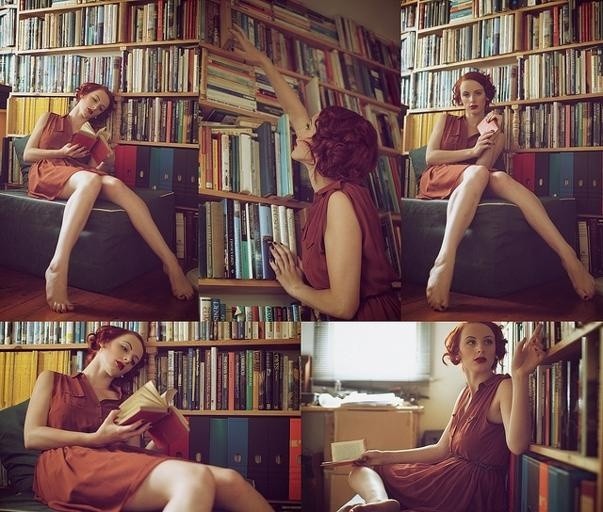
left=346, top=320, right=550, bottom=511
left=415, top=70, right=596, bottom=312
left=226, top=20, right=404, bottom=321
left=19, top=82, right=196, bottom=315
left=21, top=325, right=276, bottom=511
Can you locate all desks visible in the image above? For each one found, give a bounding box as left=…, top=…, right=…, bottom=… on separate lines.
left=301, top=404, right=425, bottom=511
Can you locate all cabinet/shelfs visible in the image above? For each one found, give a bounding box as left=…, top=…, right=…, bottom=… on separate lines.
left=199, top=0, right=399, bottom=323
left=0, top=322, right=301, bottom=512
left=403, top=0, right=602, bottom=298
left=400, top=0, right=419, bottom=126
left=6, top=0, right=124, bottom=138
left=113, top=0, right=200, bottom=262
left=495, top=321, right=601, bottom=512
left=0, top=0, right=20, bottom=88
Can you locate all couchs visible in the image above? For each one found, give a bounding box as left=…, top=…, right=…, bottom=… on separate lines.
left=0, top=491, right=64, bottom=512
left=401, top=197, right=575, bottom=299
left=1, top=189, right=175, bottom=294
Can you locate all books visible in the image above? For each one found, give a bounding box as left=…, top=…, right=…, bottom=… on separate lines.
left=508, top=319, right=601, bottom=511
left=400, top=0, right=603, bottom=282
left=69, top=121, right=113, bottom=169
left=113, top=381, right=191, bottom=451
left=475, top=114, right=501, bottom=142
left=198, top=1, right=402, bottom=321
left=319, top=437, right=368, bottom=476
left=0, top=320, right=302, bottom=512
left=0, top=2, right=198, bottom=270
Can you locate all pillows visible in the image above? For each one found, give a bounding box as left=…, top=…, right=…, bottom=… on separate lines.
left=409, top=146, right=427, bottom=182
left=11, top=135, right=33, bottom=191
left=0, top=394, right=41, bottom=495
